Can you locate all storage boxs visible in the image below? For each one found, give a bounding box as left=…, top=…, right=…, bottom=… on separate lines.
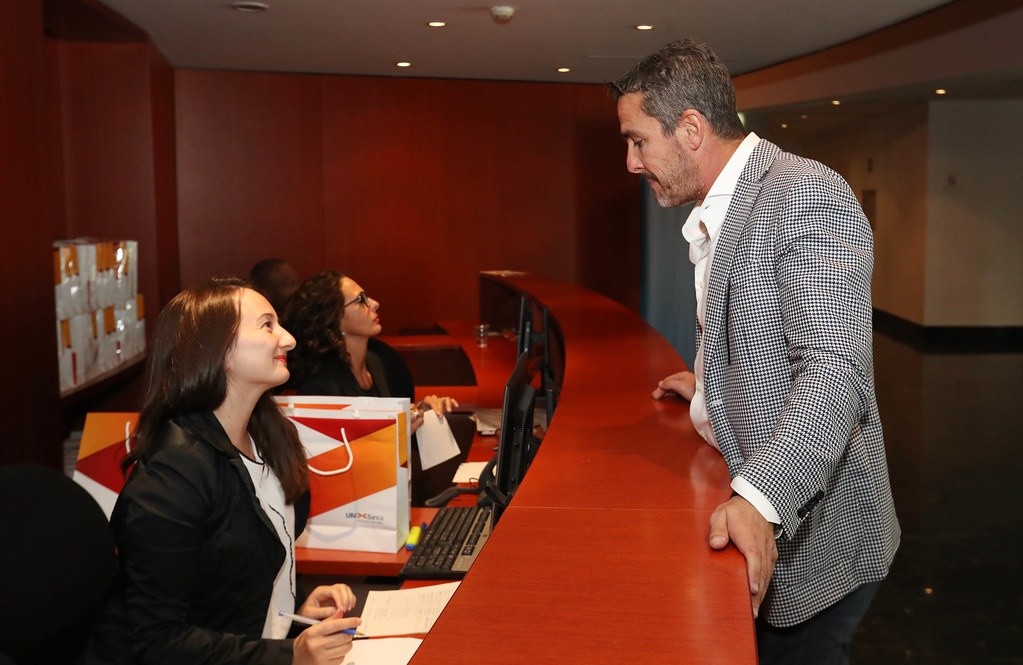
left=53, top=235, right=145, bottom=388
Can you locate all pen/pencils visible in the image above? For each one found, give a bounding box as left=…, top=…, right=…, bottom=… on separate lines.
left=279, top=612, right=364, bottom=636
left=414, top=401, right=422, bottom=409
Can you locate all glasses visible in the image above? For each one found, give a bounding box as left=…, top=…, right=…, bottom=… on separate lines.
left=342, top=291, right=367, bottom=310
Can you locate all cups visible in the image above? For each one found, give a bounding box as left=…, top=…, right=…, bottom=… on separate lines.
left=474, top=324, right=489, bottom=348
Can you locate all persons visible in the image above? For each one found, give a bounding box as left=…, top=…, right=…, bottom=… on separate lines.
left=101, top=276, right=362, bottom=665
left=605, top=37, right=902, bottom=665
left=247, top=258, right=459, bottom=508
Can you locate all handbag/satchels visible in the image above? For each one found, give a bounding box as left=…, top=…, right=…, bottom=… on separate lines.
left=269, top=396, right=412, bottom=555
left=73, top=412, right=140, bottom=523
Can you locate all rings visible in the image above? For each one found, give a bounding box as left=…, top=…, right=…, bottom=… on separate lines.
left=411, top=408, right=420, bottom=419
